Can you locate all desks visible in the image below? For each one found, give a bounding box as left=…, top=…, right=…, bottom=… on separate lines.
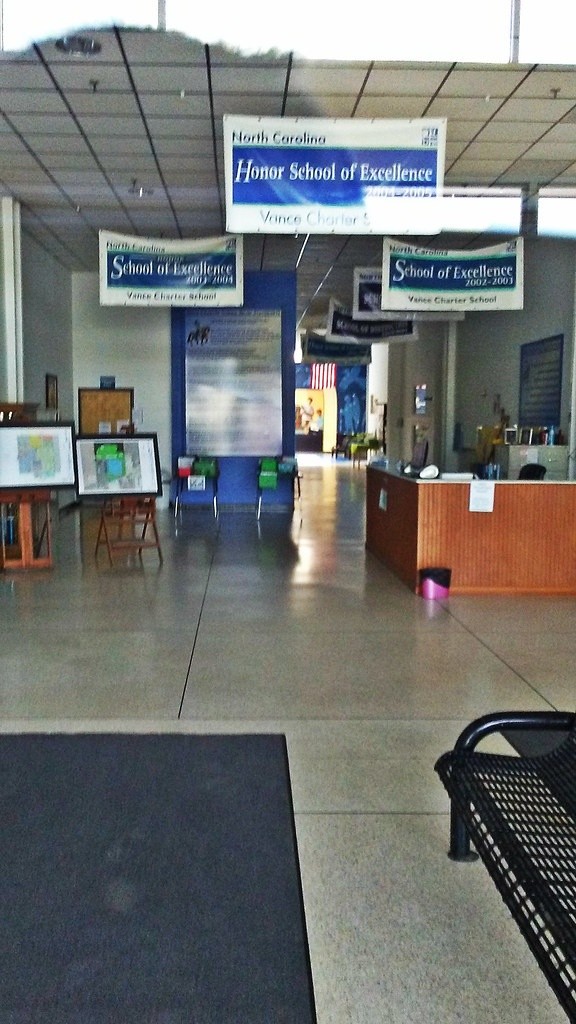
left=352, top=443, right=381, bottom=468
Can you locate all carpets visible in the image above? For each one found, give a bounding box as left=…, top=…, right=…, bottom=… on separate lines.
left=0, top=732, right=322, bottom=1023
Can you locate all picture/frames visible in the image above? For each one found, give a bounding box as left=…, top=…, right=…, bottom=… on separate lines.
left=505, top=428, right=518, bottom=444
left=46, top=372, right=58, bottom=413
left=518, top=429, right=533, bottom=446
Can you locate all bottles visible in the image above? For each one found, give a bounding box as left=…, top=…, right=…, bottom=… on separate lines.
left=538, top=425, right=565, bottom=445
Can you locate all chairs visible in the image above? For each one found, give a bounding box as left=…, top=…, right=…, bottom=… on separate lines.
left=518, top=463, right=547, bottom=482
left=331, top=436, right=351, bottom=458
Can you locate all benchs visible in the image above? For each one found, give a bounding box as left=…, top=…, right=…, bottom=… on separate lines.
left=435, top=709, right=576, bottom=1024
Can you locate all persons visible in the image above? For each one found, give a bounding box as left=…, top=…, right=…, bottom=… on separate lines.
left=317, top=410, right=323, bottom=431
left=299, top=397, right=314, bottom=423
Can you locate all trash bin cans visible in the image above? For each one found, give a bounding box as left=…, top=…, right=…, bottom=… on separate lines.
left=419, top=567, right=452, bottom=600
